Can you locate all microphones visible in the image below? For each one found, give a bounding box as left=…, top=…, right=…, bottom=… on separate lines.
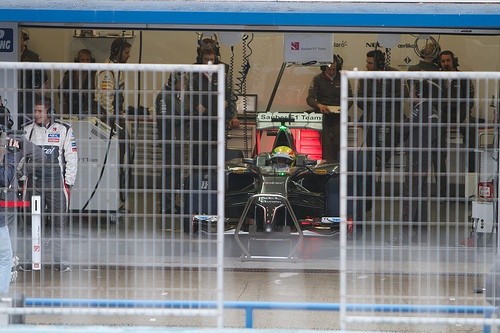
left=6, top=130, right=27, bottom=134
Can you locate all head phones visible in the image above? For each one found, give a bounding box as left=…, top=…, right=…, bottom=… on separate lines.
left=437, top=55, right=460, bottom=68
left=110, top=40, right=124, bottom=56
left=196, top=51, right=221, bottom=64
left=22, top=29, right=29, bottom=41
left=321, top=54, right=343, bottom=71
left=168, top=75, right=177, bottom=87
left=74, top=55, right=95, bottom=63
left=376, top=51, right=384, bottom=67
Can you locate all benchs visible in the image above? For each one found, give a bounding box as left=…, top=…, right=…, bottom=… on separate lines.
left=122, top=121, right=469, bottom=187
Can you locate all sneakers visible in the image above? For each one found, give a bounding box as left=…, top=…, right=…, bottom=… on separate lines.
left=51, top=259, right=72, bottom=276
left=18, top=257, right=41, bottom=274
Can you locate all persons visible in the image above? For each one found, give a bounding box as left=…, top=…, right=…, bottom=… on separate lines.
left=433, top=50, right=476, bottom=204
left=19, top=98, right=78, bottom=272
left=407, top=42, right=441, bottom=87
left=0, top=96, right=47, bottom=298
left=94, top=38, right=132, bottom=213
left=156, top=72, right=190, bottom=214
left=357, top=50, right=410, bottom=163
left=57, top=49, right=102, bottom=114
left=395, top=83, right=438, bottom=230
left=306, top=54, right=353, bottom=162
left=187, top=38, right=233, bottom=190
left=18, top=30, right=49, bottom=130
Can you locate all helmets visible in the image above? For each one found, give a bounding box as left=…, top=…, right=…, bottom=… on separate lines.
left=271, top=145, right=296, bottom=171
